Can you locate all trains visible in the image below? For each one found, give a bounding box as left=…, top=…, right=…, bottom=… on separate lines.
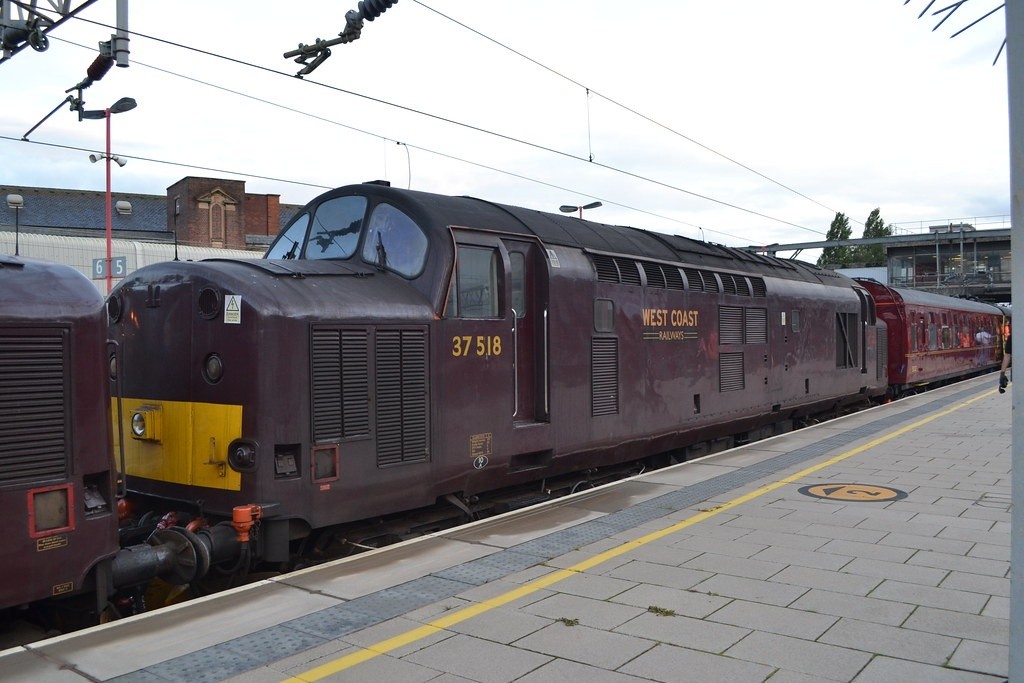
left=0, top=178, right=1013, bottom=633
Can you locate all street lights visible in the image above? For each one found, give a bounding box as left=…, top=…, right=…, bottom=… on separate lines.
left=559, top=201, right=603, bottom=220
left=80, top=96, right=138, bottom=301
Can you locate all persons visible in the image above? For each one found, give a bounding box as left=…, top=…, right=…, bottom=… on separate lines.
left=999, top=334, right=1012, bottom=388
left=976, top=326, right=997, bottom=346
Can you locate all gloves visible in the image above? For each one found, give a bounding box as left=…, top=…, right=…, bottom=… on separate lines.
left=1000, top=376, right=1008, bottom=388
